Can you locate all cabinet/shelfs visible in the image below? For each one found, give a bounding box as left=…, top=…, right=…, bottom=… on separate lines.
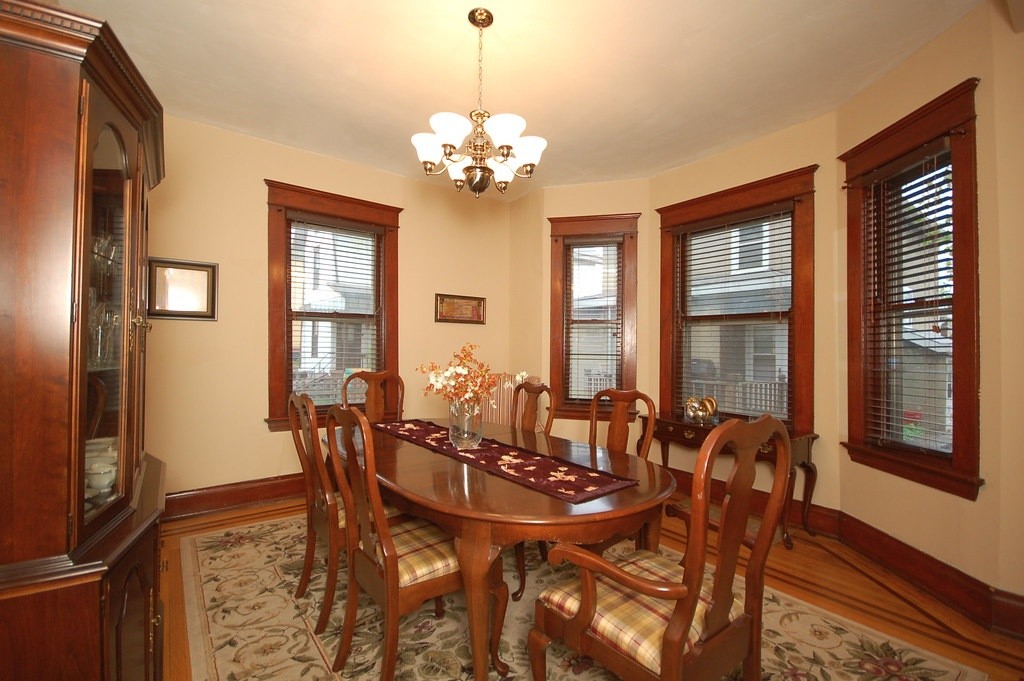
left=0, top=0, right=165, bottom=681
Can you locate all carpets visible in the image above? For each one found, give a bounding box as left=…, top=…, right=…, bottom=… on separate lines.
left=179, top=511, right=990, bottom=681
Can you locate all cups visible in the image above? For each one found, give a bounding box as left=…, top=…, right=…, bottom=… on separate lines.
left=85, top=463, right=117, bottom=494
left=86, top=230, right=125, bottom=366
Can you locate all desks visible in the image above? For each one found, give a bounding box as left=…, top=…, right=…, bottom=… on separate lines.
left=636, top=411, right=820, bottom=550
left=321, top=416, right=677, bottom=681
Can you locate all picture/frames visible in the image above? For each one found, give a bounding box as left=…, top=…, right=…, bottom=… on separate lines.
left=435, top=293, right=487, bottom=324
left=147, top=255, right=219, bottom=321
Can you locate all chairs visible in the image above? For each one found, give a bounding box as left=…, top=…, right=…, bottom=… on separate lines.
left=288, top=369, right=656, bottom=681
left=529, top=414, right=792, bottom=681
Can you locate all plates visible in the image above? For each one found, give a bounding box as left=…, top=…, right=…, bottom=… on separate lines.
left=83, top=437, right=117, bottom=468
left=84, top=488, right=100, bottom=500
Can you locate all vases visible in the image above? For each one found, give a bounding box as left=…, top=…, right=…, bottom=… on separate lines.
left=449, top=402, right=483, bottom=449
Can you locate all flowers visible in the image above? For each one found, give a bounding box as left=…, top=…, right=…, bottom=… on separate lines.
left=413, top=342, right=528, bottom=440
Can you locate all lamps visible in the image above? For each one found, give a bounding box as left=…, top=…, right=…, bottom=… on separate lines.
left=411, top=7, right=548, bottom=199
